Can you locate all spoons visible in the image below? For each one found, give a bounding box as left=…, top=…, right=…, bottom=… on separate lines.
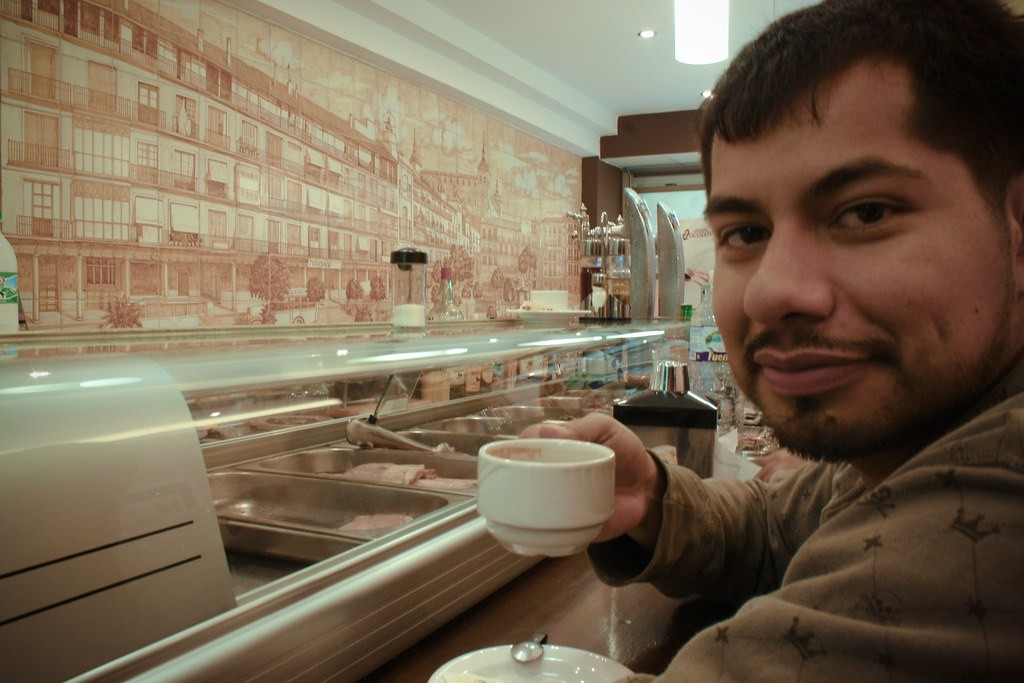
left=512, top=632, right=548, bottom=664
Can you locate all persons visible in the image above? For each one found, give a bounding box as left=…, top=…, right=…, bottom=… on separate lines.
left=519, top=0, right=1024, bottom=683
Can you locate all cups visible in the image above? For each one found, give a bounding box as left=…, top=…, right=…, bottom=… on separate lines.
left=476, top=437, right=616, bottom=557
left=529, top=290, right=569, bottom=312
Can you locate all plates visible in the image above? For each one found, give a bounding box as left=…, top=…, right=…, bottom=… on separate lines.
left=507, top=307, right=592, bottom=328
left=427, top=641, right=640, bottom=683
